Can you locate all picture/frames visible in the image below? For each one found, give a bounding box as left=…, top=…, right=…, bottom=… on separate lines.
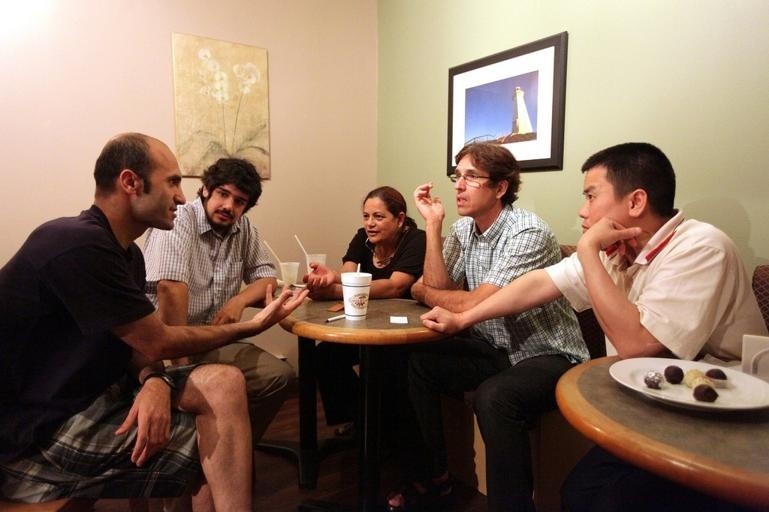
left=444, top=31, right=570, bottom=175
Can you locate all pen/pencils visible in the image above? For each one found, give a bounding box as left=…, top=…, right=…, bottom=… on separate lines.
left=325, top=315, right=346, bottom=322
left=422, top=196, right=431, bottom=205
left=288, top=283, right=308, bottom=287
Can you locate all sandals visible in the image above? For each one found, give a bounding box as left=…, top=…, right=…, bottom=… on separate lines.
left=385, top=470, right=454, bottom=509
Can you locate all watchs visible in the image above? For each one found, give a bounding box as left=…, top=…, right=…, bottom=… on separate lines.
left=140, top=371, right=177, bottom=391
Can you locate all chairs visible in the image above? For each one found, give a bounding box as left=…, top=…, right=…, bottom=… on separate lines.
left=752, top=264, right=769, bottom=334
left=139, top=276, right=308, bottom=494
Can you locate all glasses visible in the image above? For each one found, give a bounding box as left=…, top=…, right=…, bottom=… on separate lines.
left=451, top=171, right=496, bottom=183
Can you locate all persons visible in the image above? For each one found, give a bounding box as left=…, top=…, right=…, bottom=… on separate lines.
left=302, top=185, right=425, bottom=438
left=141, top=159, right=294, bottom=453
left=419, top=141, right=769, bottom=511
left=384, top=141, right=591, bottom=512
left=1, top=132, right=310, bottom=512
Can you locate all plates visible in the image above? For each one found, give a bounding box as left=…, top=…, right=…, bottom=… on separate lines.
left=607, top=354, right=768, bottom=413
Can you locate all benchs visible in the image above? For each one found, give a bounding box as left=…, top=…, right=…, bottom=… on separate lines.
left=440, top=237, right=610, bottom=509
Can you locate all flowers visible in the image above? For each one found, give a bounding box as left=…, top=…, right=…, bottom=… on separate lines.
left=184, top=43, right=270, bottom=182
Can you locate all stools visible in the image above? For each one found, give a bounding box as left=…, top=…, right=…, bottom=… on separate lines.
left=2, top=494, right=101, bottom=510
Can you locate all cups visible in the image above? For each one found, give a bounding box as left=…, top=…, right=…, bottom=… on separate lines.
left=305, top=253, right=327, bottom=276
left=280, top=262, right=301, bottom=284
left=340, top=272, right=372, bottom=322
